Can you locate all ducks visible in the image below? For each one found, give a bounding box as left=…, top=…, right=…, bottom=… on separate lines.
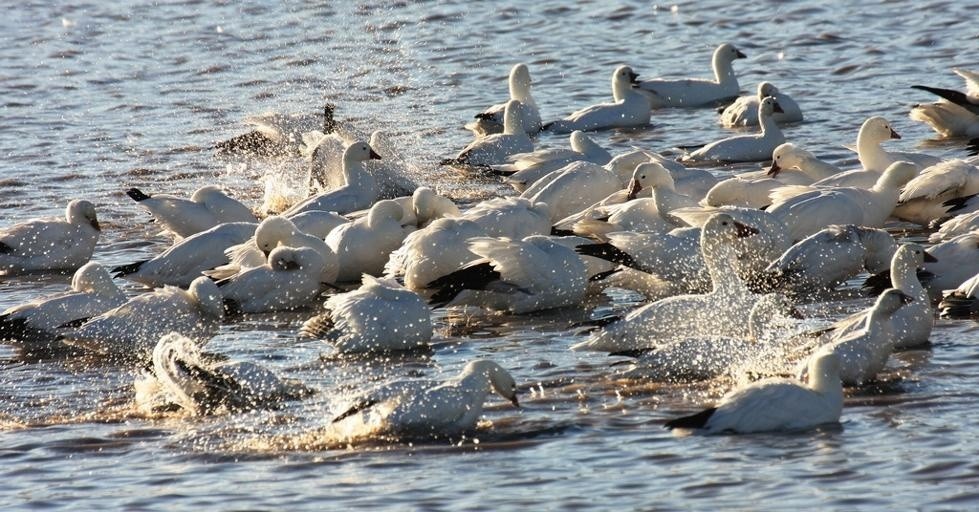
left=2, top=43, right=979, bottom=446
